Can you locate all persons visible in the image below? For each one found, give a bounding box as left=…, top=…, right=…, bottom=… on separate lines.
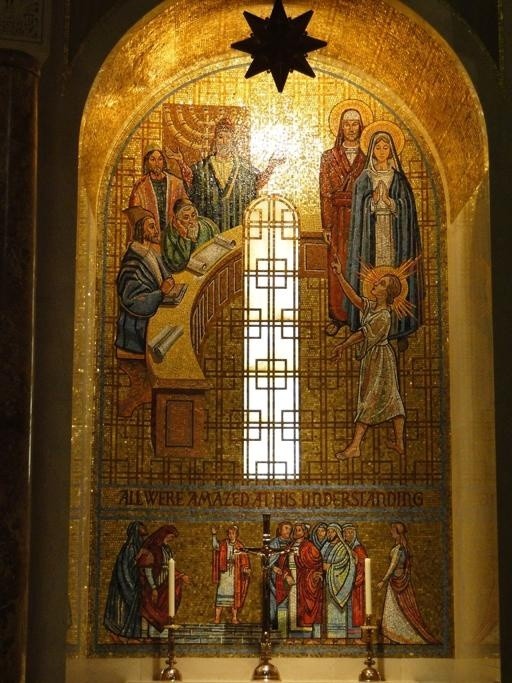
left=135, top=523, right=191, bottom=648
left=317, top=97, right=375, bottom=337
left=264, top=520, right=369, bottom=646
left=103, top=520, right=149, bottom=648
left=161, top=196, right=219, bottom=272
left=209, top=523, right=252, bottom=625
left=339, top=117, right=427, bottom=354
left=126, top=141, right=188, bottom=248
left=329, top=253, right=409, bottom=462
left=113, top=203, right=175, bottom=354
left=186, top=113, right=287, bottom=231
left=373, top=521, right=442, bottom=645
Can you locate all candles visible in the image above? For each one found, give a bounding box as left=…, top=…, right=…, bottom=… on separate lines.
left=364, top=557, right=373, bottom=616
left=168, top=557, right=176, bottom=618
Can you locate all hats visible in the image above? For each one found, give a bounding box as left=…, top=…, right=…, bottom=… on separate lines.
left=143, top=144, right=162, bottom=160
left=214, top=119, right=235, bottom=138
left=122, top=205, right=155, bottom=226
left=342, top=110, right=361, bottom=121
left=173, top=198, right=193, bottom=214
